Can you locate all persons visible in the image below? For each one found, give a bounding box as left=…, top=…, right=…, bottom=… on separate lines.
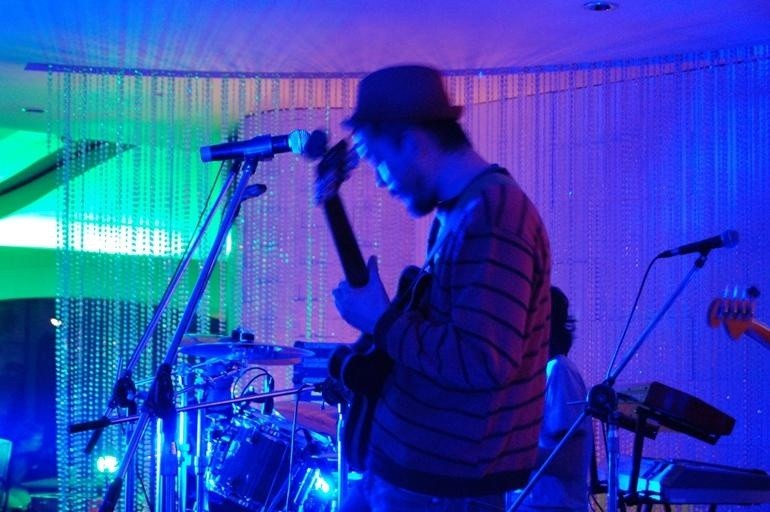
left=503, top=284, right=592, bottom=512
left=330, top=60, right=550, bottom=512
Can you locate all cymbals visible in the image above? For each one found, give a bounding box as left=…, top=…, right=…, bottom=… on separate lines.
left=170, top=332, right=315, bottom=364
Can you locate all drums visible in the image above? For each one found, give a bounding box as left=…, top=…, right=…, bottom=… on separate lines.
left=203, top=406, right=363, bottom=512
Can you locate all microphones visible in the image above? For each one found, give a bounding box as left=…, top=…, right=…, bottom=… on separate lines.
left=302, top=427, right=332, bottom=475
left=229, top=184, right=266, bottom=202
left=200, top=129, right=311, bottom=162
left=657, top=229, right=739, bottom=258
left=262, top=378, right=275, bottom=417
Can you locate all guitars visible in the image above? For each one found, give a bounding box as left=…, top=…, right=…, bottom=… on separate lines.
left=314, top=138, right=429, bottom=471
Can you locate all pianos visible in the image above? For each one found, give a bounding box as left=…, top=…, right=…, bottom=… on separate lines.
left=611, top=382, right=735, bottom=446
left=596, top=454, right=768, bottom=504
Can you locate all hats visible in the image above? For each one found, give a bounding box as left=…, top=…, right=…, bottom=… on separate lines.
left=551, top=287, right=568, bottom=320
left=341, top=66, right=463, bottom=126
